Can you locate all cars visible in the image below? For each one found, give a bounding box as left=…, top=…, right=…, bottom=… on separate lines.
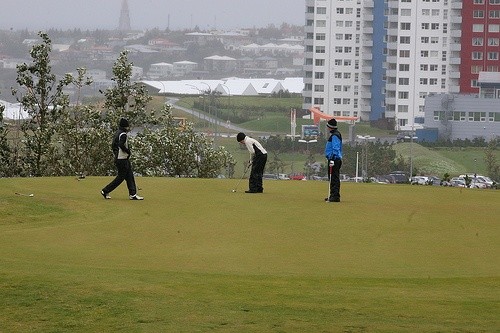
left=396, top=132, right=418, bottom=143
left=263, top=173, right=331, bottom=181
left=340, top=170, right=500, bottom=190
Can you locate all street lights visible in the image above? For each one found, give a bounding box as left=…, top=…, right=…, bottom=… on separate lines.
left=298, top=139, right=318, bottom=181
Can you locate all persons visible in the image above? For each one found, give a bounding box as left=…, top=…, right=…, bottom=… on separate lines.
left=323, top=118, right=344, bottom=203
left=236, top=132, right=269, bottom=194
left=99, top=117, right=145, bottom=201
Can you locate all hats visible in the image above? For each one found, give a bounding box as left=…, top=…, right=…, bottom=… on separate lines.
left=237, top=133, right=246, bottom=142
left=119, top=118, right=129, bottom=128
left=327, top=119, right=337, bottom=129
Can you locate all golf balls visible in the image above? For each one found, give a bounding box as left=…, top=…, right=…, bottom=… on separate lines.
left=232, top=190, right=234, bottom=192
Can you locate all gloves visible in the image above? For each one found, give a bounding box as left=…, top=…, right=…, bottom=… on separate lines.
left=329, top=160, right=334, bottom=166
left=250, top=161, right=253, bottom=165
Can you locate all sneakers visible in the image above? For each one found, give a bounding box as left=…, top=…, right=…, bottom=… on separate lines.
left=99, top=189, right=111, bottom=199
left=256, top=189, right=264, bottom=193
left=325, top=197, right=340, bottom=202
left=245, top=190, right=257, bottom=193
left=129, top=194, right=144, bottom=200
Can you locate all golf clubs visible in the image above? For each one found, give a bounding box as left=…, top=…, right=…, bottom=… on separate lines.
left=325, top=164, right=332, bottom=204
left=104, top=158, right=143, bottom=191
left=234, top=165, right=251, bottom=193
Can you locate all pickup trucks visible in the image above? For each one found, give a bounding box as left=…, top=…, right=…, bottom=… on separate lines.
left=358, top=135, right=375, bottom=140
